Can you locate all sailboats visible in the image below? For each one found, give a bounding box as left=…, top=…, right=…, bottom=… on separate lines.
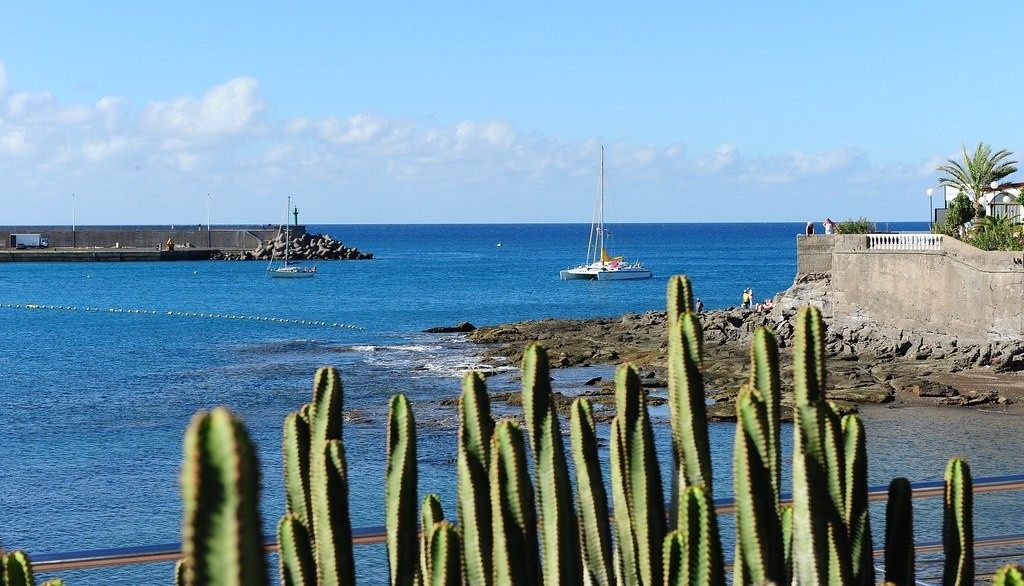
left=558, top=145, right=653, bottom=281
left=265, top=196, right=318, bottom=278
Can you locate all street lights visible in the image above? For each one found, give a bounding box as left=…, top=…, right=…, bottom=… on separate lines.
left=990, top=181, right=999, bottom=229
left=1002, top=195, right=1010, bottom=221
left=926, top=187, right=935, bottom=233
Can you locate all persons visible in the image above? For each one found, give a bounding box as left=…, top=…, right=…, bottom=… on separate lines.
left=742, top=290, right=750, bottom=309
left=805, top=221, right=815, bottom=237
left=823, top=218, right=836, bottom=234
left=755, top=299, right=773, bottom=316
left=747, top=288, right=754, bottom=310
left=155, top=243, right=162, bottom=251
left=696, top=298, right=702, bottom=314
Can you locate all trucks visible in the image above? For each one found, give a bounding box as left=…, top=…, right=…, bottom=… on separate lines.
left=9, top=233, right=49, bottom=249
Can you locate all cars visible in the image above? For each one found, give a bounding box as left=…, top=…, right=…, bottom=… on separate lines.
left=16, top=242, right=26, bottom=250
